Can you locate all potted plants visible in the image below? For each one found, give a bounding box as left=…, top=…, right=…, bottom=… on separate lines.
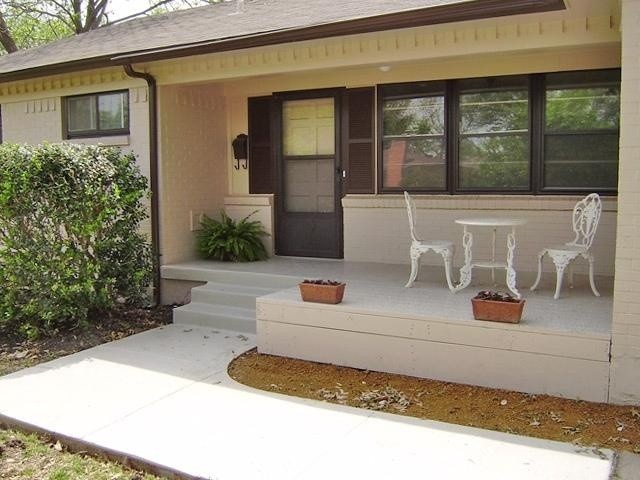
left=297, top=278, right=346, bottom=305
left=471, top=289, right=526, bottom=324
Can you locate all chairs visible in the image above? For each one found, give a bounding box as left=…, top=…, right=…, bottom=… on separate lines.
left=528, top=193, right=602, bottom=300
left=402, top=190, right=456, bottom=294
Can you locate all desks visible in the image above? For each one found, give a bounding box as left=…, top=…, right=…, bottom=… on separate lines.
left=451, top=215, right=532, bottom=300
